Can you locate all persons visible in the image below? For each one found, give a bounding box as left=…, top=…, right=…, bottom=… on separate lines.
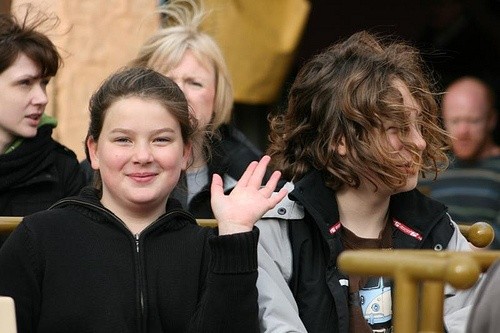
left=412, top=77, right=499, bottom=254
left=1, top=13, right=84, bottom=247
left=78, top=0, right=264, bottom=219
left=0, top=67, right=289, bottom=333
left=253, top=32, right=485, bottom=333
